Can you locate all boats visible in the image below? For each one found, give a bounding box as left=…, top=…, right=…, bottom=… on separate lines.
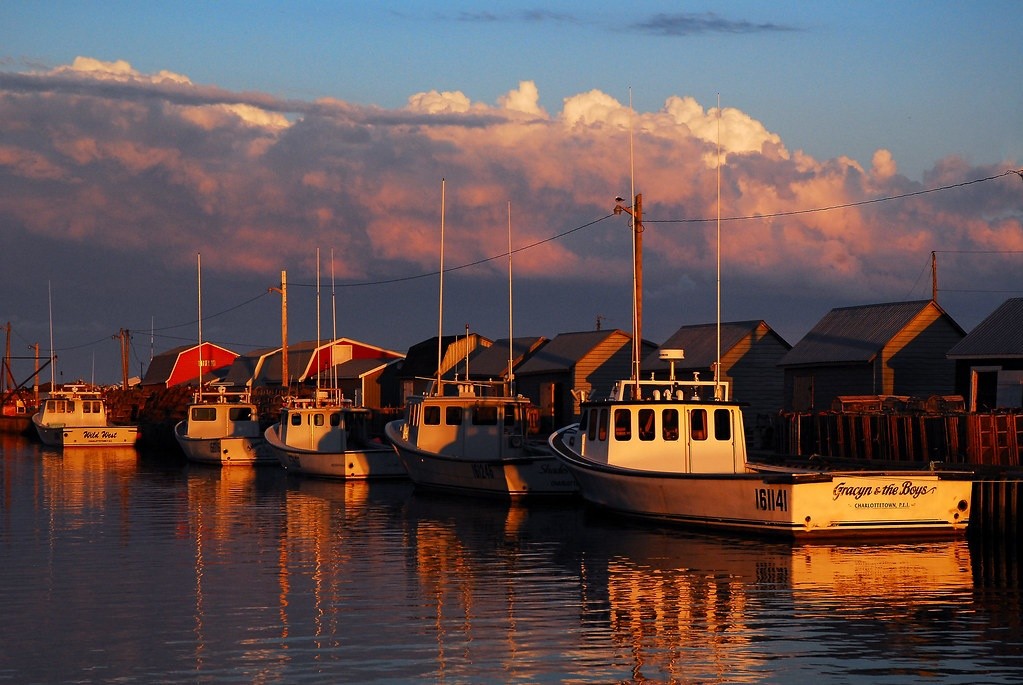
left=174, top=253, right=279, bottom=465
left=548, top=86, right=975, bottom=539
left=32, top=278, right=142, bottom=448
left=264, top=247, right=413, bottom=479
left=384, top=177, right=582, bottom=502
